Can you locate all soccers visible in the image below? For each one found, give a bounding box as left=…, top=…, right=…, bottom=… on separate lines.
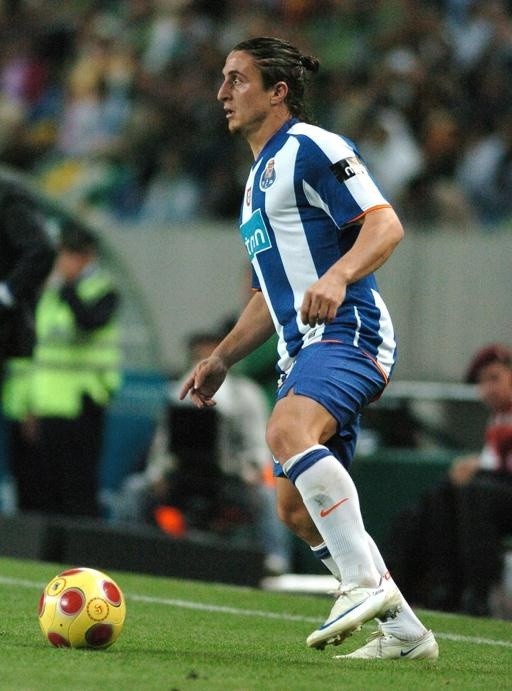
left=38, top=567, right=126, bottom=651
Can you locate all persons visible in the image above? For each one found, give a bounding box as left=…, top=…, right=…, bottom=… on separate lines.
left=134, top=332, right=294, bottom=583
left=0, top=221, right=121, bottom=518
left=174, top=36, right=441, bottom=667
left=428, top=344, right=512, bottom=615
left=2, top=1, right=511, bottom=384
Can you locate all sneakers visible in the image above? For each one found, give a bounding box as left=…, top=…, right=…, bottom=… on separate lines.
left=305, top=577, right=401, bottom=651
left=333, top=630, right=439, bottom=660
